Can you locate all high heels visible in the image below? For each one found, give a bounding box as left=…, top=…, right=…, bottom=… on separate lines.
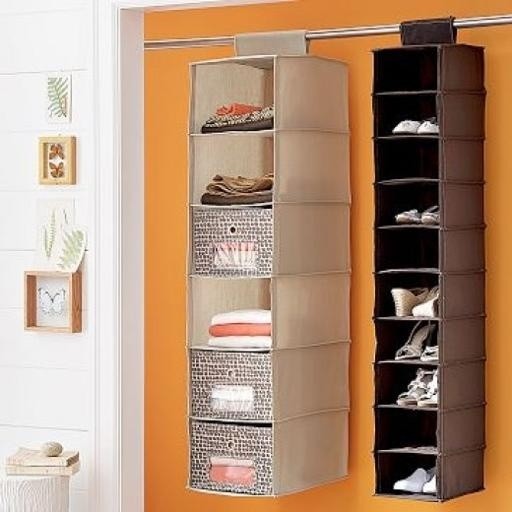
left=391, top=284, right=439, bottom=407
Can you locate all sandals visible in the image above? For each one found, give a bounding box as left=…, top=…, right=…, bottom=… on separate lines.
left=395, top=206, right=440, bottom=224
left=392, top=117, right=439, bottom=134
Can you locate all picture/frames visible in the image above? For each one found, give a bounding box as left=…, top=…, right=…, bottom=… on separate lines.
left=23, top=270, right=82, bottom=333
left=39, top=135, right=77, bottom=186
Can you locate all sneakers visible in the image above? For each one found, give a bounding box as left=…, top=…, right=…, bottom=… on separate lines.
left=393, top=468, right=436, bottom=494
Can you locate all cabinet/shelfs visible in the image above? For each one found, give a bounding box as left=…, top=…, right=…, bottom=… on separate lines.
left=370, top=15, right=486, bottom=502
left=185, top=28, right=351, bottom=499
